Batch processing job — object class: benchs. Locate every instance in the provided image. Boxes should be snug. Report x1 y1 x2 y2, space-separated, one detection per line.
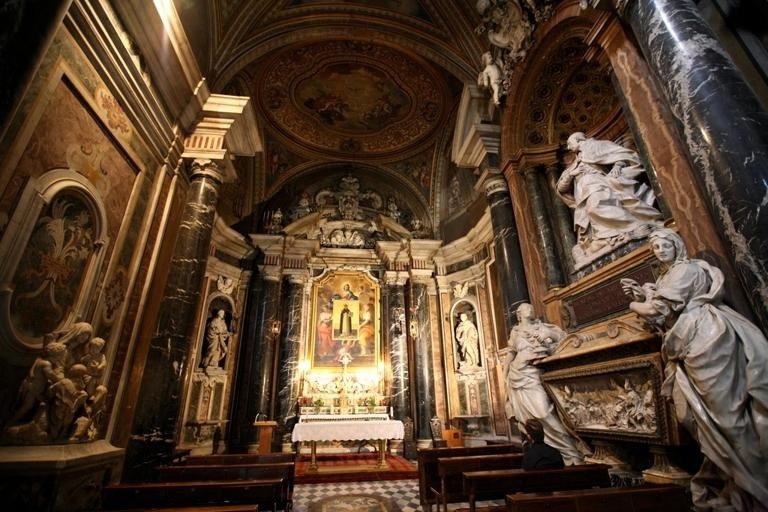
417 441 687 512
97 451 296 512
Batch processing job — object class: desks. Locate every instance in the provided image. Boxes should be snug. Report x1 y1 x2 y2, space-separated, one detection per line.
291 413 405 473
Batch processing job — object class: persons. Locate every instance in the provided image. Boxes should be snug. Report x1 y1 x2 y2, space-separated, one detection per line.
200 307 234 371
516 418 569 471
211 425 222 455
46 362 88 439
340 302 354 337
4 342 67 439
333 282 358 300
43 321 94 373
355 302 376 356
618 226 768 510
334 339 357 360
502 301 592 467
318 302 337 361
72 336 108 444
455 310 479 368
475 50 506 106
555 129 668 270
